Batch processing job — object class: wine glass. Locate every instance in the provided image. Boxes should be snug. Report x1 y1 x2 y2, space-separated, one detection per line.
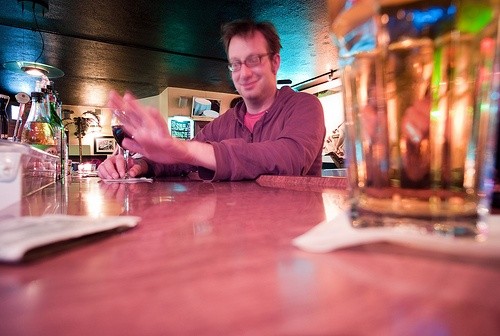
111 113 147 182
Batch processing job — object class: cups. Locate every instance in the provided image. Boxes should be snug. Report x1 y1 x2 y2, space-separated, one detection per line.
334 0 500 238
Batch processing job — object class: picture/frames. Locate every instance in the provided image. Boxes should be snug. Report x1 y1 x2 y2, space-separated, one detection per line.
94 136 117 154
191 96 222 118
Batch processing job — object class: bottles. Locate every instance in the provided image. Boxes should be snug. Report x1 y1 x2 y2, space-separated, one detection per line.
23 84 69 180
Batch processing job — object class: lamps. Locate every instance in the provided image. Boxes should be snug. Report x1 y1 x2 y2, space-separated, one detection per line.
327 69 334 81
2 0 65 79
72 116 94 164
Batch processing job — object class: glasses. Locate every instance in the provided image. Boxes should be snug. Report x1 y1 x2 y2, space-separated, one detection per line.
226 52 270 72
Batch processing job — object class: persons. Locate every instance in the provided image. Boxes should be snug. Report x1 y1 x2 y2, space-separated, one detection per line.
96 18 326 182
